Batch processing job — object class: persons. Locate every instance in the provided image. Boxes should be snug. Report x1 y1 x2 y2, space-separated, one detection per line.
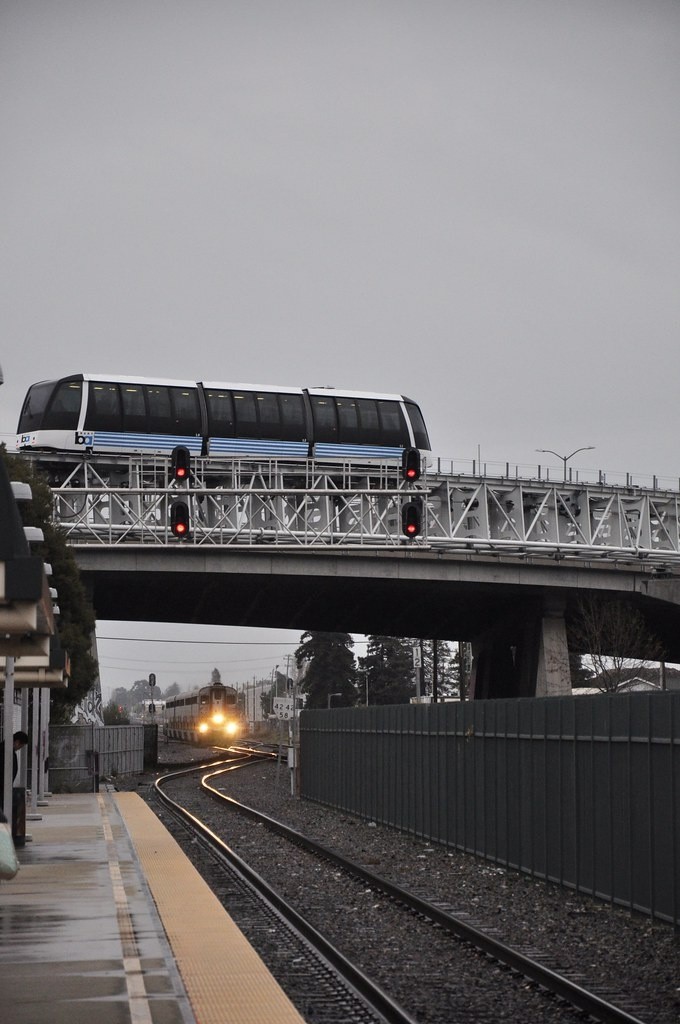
0 731 31 786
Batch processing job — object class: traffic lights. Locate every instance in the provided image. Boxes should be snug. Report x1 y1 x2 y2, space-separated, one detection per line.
401 446 420 483
403 503 422 537
170 445 192 482
119 706 123 714
171 500 189 536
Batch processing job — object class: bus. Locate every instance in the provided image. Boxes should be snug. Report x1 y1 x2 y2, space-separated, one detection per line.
15 372 435 471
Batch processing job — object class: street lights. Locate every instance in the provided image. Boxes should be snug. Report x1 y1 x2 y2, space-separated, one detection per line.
275 664 279 698
535 446 595 482
327 691 342 710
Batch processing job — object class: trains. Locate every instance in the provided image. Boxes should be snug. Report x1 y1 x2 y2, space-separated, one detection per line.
162 683 241 747
